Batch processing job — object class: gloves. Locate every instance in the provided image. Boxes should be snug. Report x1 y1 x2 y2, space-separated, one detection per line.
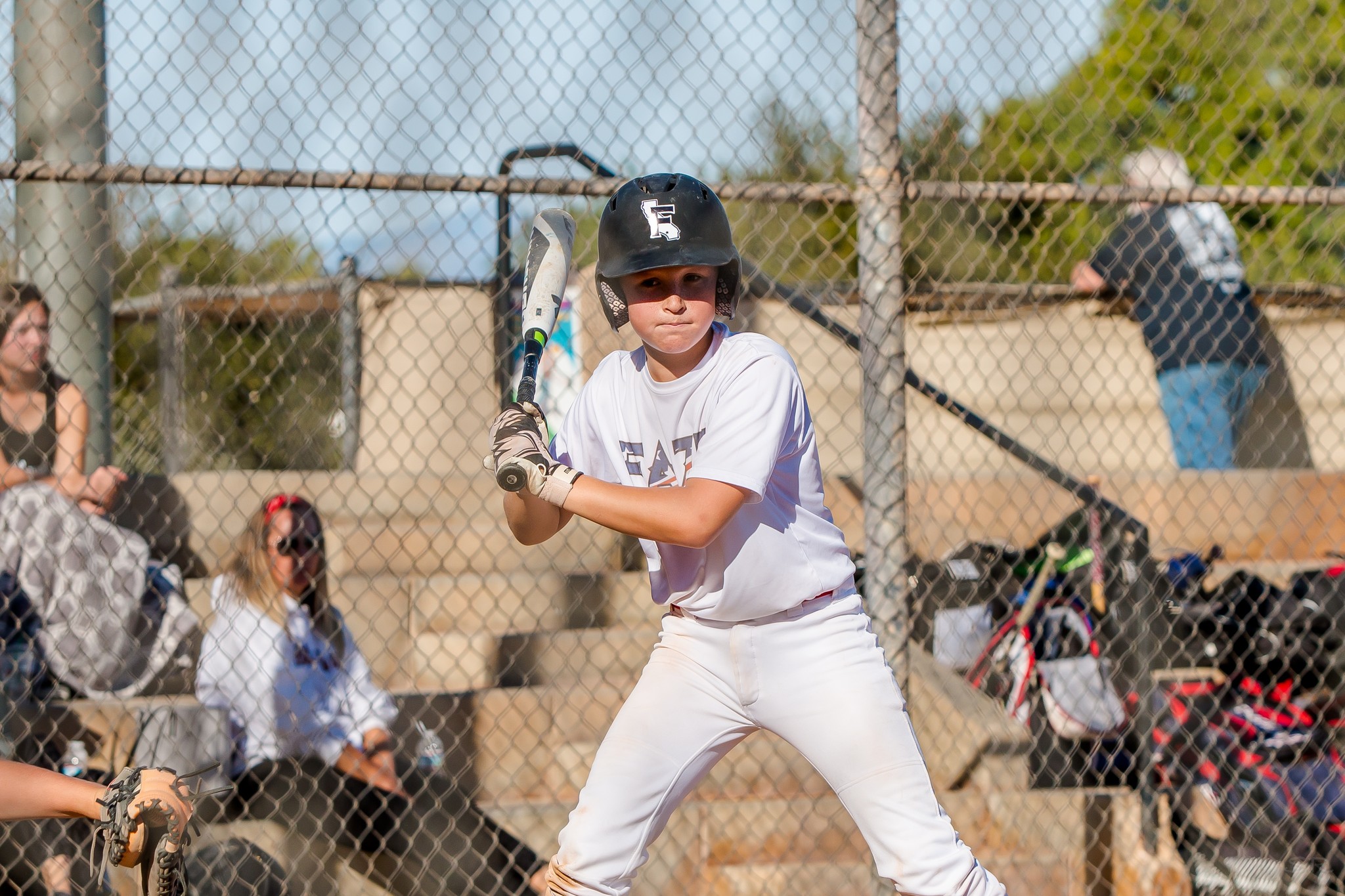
482 427 583 522
491 399 549 423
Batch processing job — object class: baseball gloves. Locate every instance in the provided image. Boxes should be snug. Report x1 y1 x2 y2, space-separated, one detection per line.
87 760 236 896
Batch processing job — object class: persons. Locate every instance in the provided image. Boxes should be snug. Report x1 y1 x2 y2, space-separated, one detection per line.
193 493 551 896
492 171 1005 896
0 280 196 896
1071 146 1280 467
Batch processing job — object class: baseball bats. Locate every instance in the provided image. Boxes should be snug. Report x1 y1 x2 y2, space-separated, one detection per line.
494 207 578 494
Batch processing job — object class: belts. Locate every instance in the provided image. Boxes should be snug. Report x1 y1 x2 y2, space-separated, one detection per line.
669 588 834 618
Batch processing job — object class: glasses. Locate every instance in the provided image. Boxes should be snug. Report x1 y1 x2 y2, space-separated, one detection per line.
270 534 325 557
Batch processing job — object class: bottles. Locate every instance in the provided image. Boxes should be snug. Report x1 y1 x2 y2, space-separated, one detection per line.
417 730 443 772
64 740 88 779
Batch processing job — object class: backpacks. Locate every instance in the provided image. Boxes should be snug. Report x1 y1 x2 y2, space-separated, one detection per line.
881 472 1336 876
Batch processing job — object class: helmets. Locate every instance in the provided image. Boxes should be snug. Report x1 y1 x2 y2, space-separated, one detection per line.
594 173 744 334
